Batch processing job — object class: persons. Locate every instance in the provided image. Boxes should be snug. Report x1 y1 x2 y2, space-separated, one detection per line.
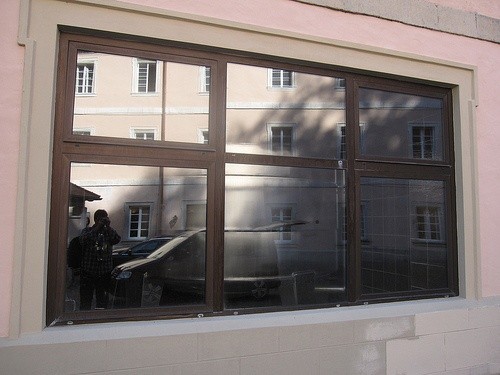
77 208 122 309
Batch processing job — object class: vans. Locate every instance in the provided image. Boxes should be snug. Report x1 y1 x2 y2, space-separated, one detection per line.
108 220 307 307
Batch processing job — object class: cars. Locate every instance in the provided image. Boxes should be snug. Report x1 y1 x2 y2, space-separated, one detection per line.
112 237 173 272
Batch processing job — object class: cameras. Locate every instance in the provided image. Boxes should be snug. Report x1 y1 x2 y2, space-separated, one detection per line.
100 217 109 224
